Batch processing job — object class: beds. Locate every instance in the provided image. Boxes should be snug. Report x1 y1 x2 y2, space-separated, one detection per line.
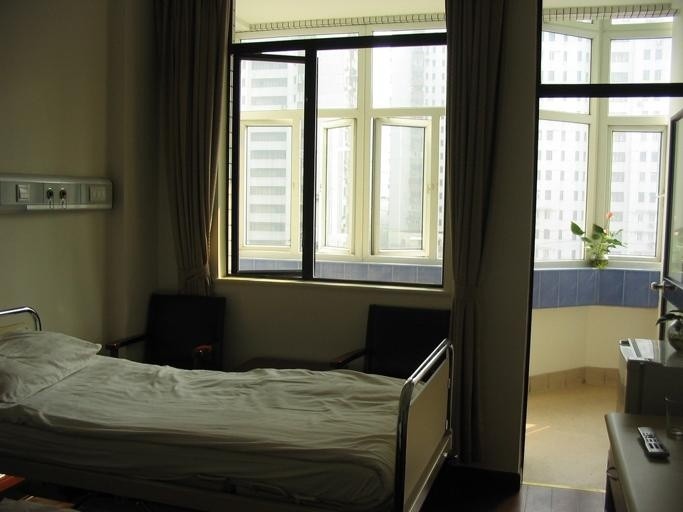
0 306 457 511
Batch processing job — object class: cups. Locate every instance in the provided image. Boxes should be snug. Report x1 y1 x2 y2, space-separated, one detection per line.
664 393 682 434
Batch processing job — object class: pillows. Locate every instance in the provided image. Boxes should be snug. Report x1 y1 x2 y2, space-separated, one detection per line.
0 329 103 409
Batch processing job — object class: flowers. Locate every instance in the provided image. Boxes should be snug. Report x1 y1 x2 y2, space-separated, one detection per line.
570 210 630 254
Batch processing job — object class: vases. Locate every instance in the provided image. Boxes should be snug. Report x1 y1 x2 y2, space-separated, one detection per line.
588 251 609 270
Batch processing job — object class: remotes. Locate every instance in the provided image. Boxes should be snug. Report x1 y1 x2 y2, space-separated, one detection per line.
637 426 669 458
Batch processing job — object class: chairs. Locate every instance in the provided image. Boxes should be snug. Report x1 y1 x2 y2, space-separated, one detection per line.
105 292 227 371
328 304 451 384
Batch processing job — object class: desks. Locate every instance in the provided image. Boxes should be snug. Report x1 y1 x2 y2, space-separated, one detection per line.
603 412 682 512
